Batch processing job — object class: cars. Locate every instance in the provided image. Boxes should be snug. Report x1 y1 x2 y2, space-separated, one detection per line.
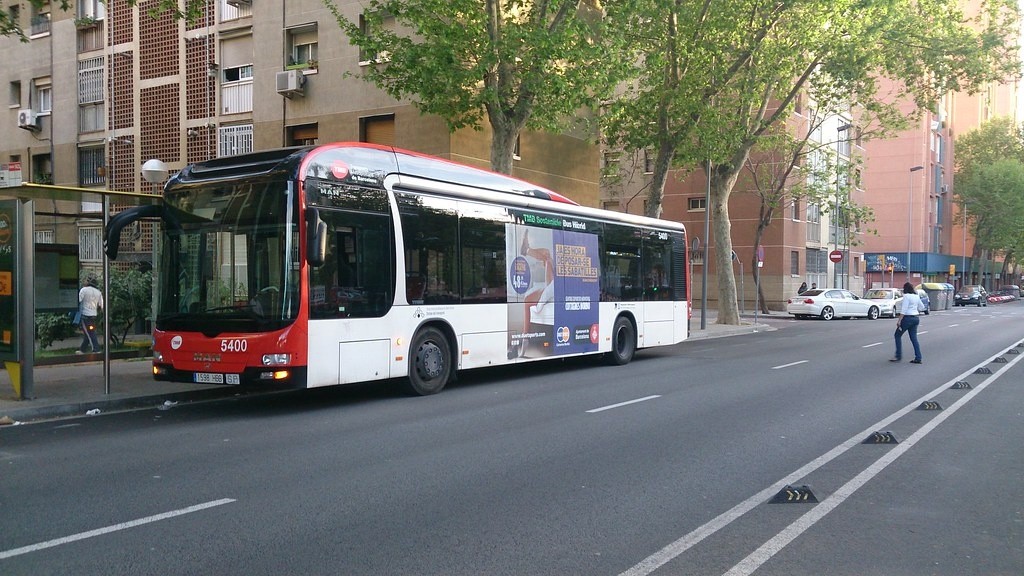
914 289 932 315
787 288 882 320
862 287 903 318
955 285 989 307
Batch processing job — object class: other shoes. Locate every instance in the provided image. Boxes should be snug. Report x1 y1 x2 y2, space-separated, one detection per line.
889 356 899 362
92 350 101 353
76 350 86 355
910 358 921 363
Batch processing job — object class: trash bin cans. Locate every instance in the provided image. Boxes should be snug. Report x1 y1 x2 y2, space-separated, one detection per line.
915 283 955 311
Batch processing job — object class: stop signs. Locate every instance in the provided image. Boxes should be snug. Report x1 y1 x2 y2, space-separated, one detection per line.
830 250 842 263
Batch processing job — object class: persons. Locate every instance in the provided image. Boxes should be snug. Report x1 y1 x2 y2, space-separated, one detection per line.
798 282 807 294
518 228 554 358
888 282 926 364
74 273 104 355
811 282 818 290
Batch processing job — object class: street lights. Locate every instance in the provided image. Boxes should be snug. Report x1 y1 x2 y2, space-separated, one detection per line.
907 165 925 283
833 123 853 287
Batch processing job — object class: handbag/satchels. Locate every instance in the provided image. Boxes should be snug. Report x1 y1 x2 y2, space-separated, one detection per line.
71 311 81 326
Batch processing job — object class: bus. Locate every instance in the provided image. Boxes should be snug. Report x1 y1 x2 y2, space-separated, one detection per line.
102 139 692 396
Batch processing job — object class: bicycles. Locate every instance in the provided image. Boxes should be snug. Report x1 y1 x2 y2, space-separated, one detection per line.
987 291 1015 304
